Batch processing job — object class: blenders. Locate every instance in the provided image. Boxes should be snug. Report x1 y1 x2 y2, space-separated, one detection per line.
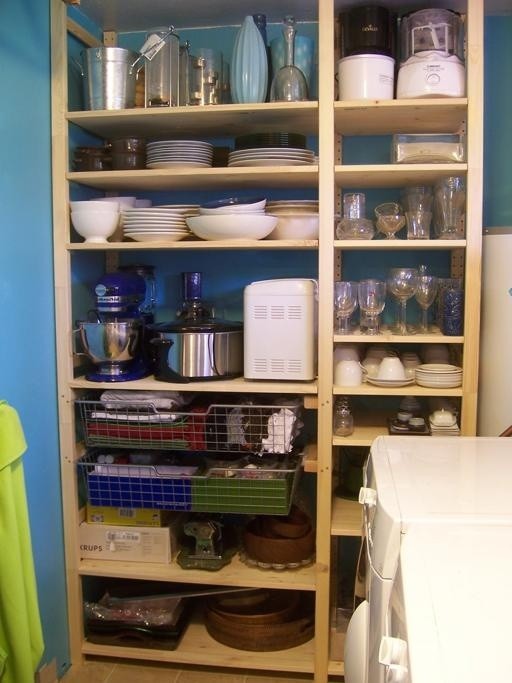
333 6 395 102
395 9 467 98
72 263 154 376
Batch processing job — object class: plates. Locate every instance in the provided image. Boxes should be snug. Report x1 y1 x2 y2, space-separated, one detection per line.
144 139 216 169
223 132 317 167
121 203 200 242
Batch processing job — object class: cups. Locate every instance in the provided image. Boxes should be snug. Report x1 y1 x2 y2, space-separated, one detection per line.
442 287 463 336
333 344 462 388
388 395 457 432
333 175 465 240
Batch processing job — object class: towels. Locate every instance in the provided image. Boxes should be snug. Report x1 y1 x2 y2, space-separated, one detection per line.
0 401 44 683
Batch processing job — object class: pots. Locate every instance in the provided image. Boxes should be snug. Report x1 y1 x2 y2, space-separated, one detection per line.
145 298 246 383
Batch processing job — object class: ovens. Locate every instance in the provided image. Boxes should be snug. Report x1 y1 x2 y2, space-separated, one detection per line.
344 434 512 683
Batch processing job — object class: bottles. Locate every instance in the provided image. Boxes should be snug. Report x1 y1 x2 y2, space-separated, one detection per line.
333 397 355 435
132 11 316 106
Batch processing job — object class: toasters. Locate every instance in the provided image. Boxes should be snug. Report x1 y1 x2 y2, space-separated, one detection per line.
240 277 317 383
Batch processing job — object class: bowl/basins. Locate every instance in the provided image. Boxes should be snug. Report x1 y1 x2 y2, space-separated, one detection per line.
242 503 314 564
70 196 154 242
186 195 323 242
73 136 146 170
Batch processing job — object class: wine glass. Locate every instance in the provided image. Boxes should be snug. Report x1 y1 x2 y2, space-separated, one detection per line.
330 265 440 334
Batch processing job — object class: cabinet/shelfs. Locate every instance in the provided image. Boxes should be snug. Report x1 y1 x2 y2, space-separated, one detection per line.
48 0 484 683
